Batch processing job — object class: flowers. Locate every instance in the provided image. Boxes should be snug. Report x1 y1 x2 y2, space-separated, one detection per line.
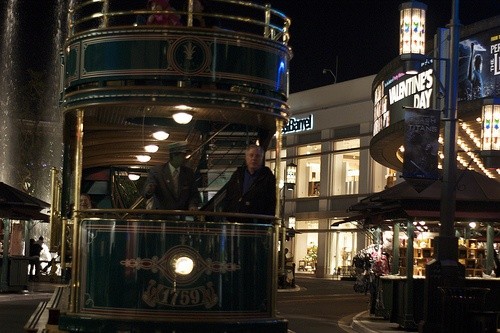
352 245 391 277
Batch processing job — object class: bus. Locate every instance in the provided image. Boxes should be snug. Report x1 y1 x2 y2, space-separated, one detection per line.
57 0 293 333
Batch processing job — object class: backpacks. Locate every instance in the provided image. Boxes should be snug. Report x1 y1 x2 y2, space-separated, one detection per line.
430 261 470 318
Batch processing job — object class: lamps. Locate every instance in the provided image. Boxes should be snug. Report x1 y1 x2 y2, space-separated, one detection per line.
171 111 193 125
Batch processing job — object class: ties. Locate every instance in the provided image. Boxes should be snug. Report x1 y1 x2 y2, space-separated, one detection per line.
173 168 179 197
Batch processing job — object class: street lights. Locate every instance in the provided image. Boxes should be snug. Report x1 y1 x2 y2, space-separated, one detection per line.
282 163 298 288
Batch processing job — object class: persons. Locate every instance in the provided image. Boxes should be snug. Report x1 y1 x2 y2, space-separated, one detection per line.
147 0 182 26
279 248 292 268
80 194 92 209
472 54 482 97
179 0 206 27
143 142 201 219
224 144 276 226
29 236 43 275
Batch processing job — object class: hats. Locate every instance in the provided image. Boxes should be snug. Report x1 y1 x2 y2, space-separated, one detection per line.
167 141 192 154
